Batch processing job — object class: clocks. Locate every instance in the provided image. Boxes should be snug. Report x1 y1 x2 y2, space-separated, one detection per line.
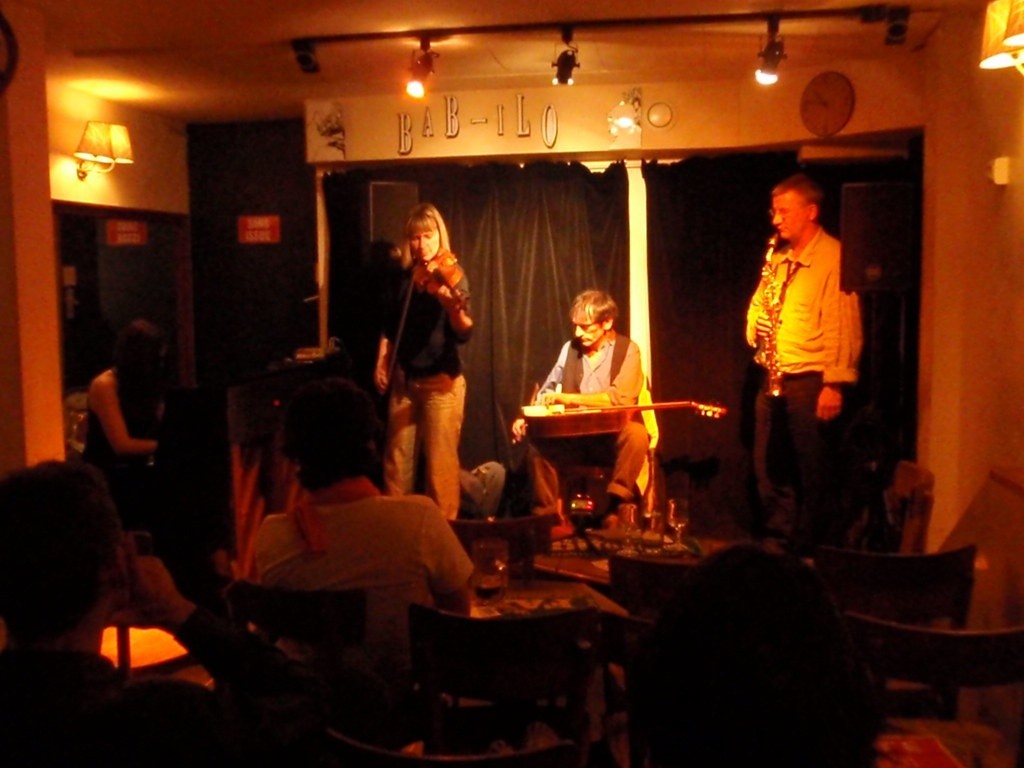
799 72 855 138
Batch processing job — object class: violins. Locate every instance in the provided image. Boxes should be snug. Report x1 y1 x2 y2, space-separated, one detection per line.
412 247 470 314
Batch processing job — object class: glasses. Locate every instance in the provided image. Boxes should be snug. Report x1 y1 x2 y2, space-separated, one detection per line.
768 204 809 216
569 320 597 330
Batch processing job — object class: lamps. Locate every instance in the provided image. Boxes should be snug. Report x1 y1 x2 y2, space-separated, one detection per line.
73 121 135 181
404 36 439 97
551 35 581 85
603 91 641 133
754 20 786 85
976 0 1024 75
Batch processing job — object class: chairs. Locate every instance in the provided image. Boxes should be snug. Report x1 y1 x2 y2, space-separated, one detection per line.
210 513 1024 768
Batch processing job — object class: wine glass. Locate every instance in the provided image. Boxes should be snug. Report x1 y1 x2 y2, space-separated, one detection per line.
668 499 689 550
618 503 639 556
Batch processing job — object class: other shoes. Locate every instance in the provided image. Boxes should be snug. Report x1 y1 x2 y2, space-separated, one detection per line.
548 520 576 541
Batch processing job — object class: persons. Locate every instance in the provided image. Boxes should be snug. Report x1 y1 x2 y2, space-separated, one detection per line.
512 290 659 528
247 378 482 687
0 461 321 768
83 321 167 474
375 205 472 520
628 544 889 768
745 174 862 542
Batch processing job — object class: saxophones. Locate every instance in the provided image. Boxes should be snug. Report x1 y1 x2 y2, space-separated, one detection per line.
753 232 785 402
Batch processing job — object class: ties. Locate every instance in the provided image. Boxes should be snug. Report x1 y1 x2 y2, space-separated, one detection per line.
776 260 801 326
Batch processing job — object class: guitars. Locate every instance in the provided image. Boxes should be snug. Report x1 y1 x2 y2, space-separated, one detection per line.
524 401 729 439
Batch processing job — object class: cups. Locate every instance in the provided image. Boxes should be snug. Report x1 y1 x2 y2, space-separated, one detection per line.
639 510 665 553
473 541 508 605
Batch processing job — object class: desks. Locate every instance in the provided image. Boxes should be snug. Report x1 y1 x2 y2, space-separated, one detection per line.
456 581 629 753
530 537 736 589
